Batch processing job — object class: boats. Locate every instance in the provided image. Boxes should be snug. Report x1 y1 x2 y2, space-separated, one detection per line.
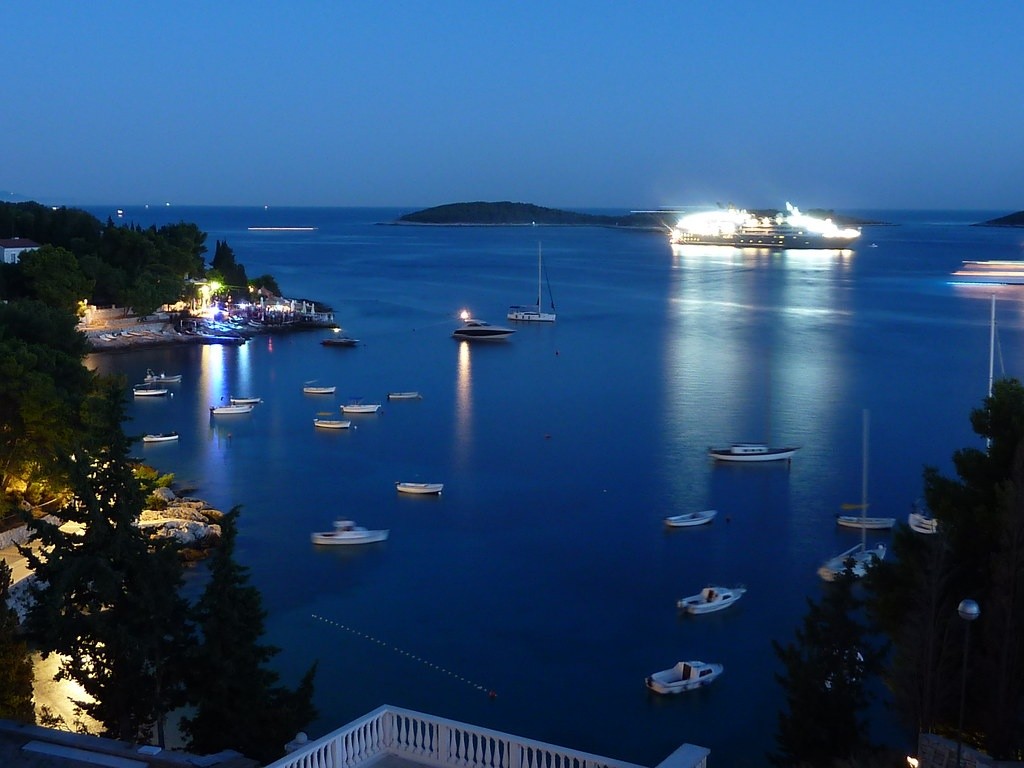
451 318 518 343
706 442 803 462
645 660 724 694
834 511 897 529
663 509 718 526
339 404 381 413
907 512 939 535
209 404 255 414
387 392 418 399
395 481 443 494
313 419 351 428
142 431 179 441
303 387 337 394
135 383 151 390
143 368 182 383
322 336 360 346
667 201 862 250
133 388 169 395
676 587 748 615
230 396 261 403
949 260 1024 284
310 520 391 545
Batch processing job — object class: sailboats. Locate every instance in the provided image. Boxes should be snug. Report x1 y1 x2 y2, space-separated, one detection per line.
817 410 887 582
507 241 557 322
